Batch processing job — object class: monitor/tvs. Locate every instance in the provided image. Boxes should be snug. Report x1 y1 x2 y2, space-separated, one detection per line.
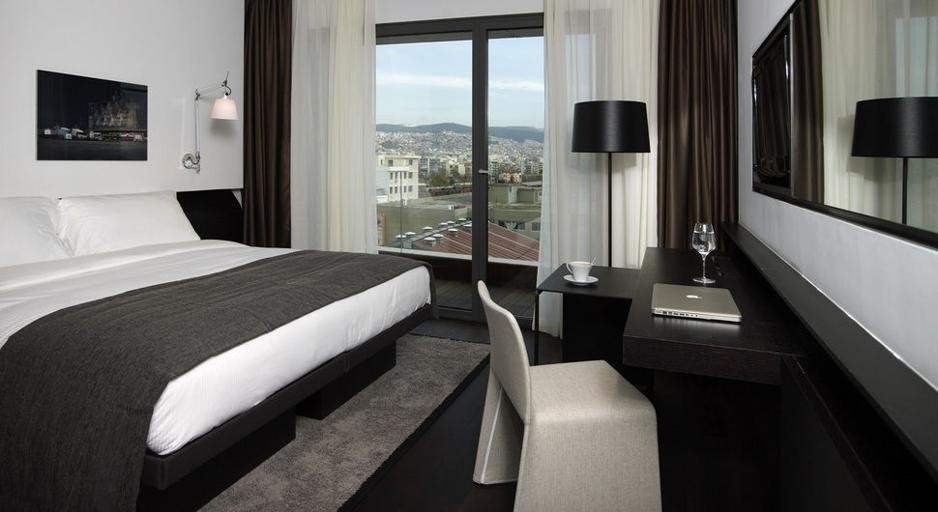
752 33 791 189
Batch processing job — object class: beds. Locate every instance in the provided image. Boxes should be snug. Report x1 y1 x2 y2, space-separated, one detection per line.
0 189 437 512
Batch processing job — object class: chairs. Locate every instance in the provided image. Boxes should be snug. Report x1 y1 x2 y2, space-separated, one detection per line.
472 280 662 512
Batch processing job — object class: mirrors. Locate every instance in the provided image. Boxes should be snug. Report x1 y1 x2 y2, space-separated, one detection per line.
752 0 938 249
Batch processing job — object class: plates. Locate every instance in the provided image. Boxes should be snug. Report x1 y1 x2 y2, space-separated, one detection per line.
563 274 600 287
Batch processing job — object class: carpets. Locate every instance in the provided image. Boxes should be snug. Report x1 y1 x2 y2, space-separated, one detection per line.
195 332 491 512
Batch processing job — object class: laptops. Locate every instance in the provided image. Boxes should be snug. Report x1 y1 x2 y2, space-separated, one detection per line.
651 283 742 324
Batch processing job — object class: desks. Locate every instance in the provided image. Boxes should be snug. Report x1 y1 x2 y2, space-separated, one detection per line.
533 246 805 386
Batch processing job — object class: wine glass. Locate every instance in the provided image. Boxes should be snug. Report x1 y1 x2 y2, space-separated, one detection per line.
692 222 717 284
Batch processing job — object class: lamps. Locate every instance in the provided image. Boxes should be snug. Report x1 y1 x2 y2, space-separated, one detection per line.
851 95 938 225
182 71 239 172
571 99 651 267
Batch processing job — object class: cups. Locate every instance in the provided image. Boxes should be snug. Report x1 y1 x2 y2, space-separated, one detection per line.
565 261 593 281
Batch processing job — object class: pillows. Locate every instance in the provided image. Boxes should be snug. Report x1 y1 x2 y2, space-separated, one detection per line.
0 196 70 267
55 191 201 258
